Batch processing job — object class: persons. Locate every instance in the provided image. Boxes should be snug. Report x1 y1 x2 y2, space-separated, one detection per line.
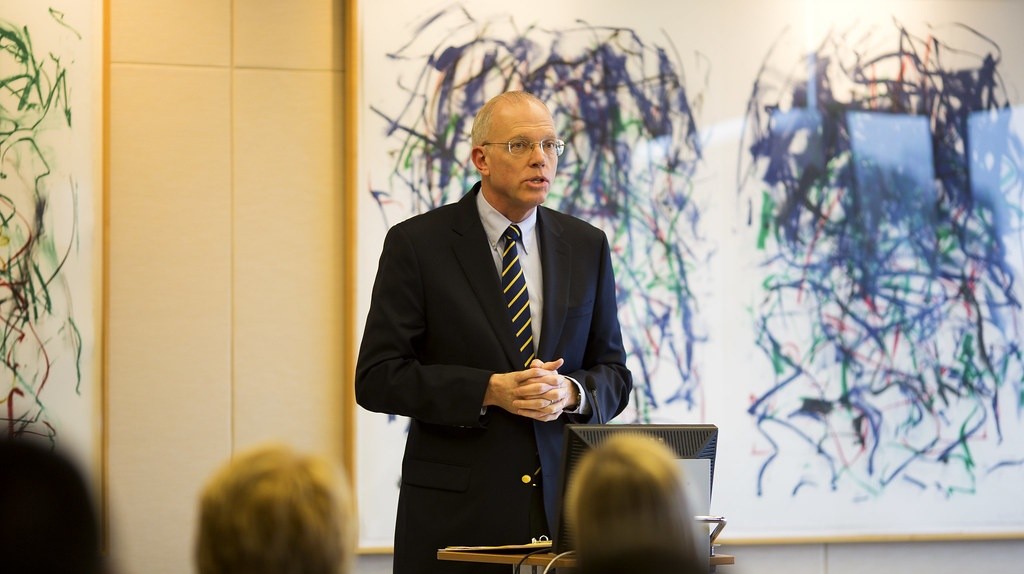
566 432 712 573
190 440 358 573
350 90 633 574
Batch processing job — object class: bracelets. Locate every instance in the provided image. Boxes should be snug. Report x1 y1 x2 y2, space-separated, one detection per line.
563 378 581 411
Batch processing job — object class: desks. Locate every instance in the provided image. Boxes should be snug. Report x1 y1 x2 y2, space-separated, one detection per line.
437 546 740 571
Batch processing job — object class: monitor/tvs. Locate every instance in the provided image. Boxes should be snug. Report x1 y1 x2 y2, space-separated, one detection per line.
551 423 719 555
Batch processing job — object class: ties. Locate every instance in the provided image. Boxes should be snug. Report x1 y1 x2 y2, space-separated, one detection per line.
499 224 537 370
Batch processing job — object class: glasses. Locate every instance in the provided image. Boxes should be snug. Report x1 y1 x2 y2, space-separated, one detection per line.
481 137 567 158
